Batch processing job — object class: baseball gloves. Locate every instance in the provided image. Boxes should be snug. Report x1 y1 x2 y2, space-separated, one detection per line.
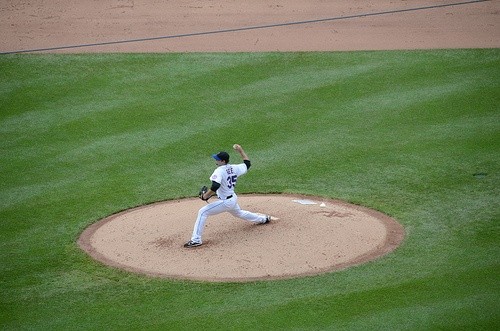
198 185 212 201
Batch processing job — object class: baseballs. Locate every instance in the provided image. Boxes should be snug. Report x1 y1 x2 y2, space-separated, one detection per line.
232 143 239 150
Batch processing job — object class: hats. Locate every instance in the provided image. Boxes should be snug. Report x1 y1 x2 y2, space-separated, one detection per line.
212 151 229 161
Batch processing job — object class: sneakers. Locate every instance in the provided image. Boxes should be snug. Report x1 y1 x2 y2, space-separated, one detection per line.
266 217 272 223
183 241 202 247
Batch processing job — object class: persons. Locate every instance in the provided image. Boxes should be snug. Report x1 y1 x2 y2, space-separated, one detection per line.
183 143 274 249
